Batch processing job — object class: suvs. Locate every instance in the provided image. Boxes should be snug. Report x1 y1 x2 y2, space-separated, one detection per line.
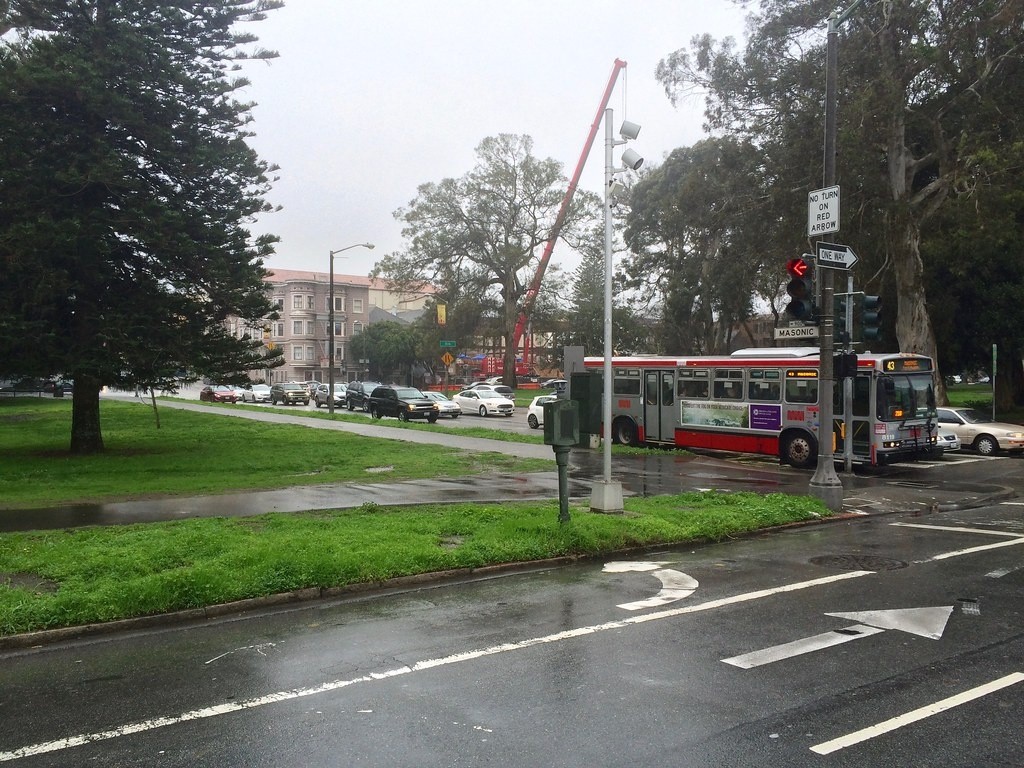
314 384 348 408
368 385 439 423
345 380 382 412
270 382 310 405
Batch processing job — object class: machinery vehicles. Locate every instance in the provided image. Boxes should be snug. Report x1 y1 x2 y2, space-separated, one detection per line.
480 58 626 380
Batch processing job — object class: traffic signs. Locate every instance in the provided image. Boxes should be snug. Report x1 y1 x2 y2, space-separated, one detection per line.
816 241 859 271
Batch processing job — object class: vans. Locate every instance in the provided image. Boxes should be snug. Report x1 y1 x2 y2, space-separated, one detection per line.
470 385 515 403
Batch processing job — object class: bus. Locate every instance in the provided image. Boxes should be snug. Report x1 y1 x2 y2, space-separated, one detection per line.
584 347 938 469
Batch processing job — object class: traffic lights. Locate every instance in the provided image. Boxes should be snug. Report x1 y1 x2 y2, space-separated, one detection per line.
785 259 812 318
863 294 884 343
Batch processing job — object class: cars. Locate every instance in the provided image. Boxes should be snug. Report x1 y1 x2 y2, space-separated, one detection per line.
460 382 490 390
14 378 73 393
451 390 515 417
200 380 321 404
540 379 558 388
920 429 961 459
527 396 559 429
419 392 461 419
916 408 1024 456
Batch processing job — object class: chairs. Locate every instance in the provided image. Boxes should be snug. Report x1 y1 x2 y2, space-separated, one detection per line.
612 378 819 406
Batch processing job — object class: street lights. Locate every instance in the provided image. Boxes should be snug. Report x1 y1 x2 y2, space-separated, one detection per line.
329 243 375 413
